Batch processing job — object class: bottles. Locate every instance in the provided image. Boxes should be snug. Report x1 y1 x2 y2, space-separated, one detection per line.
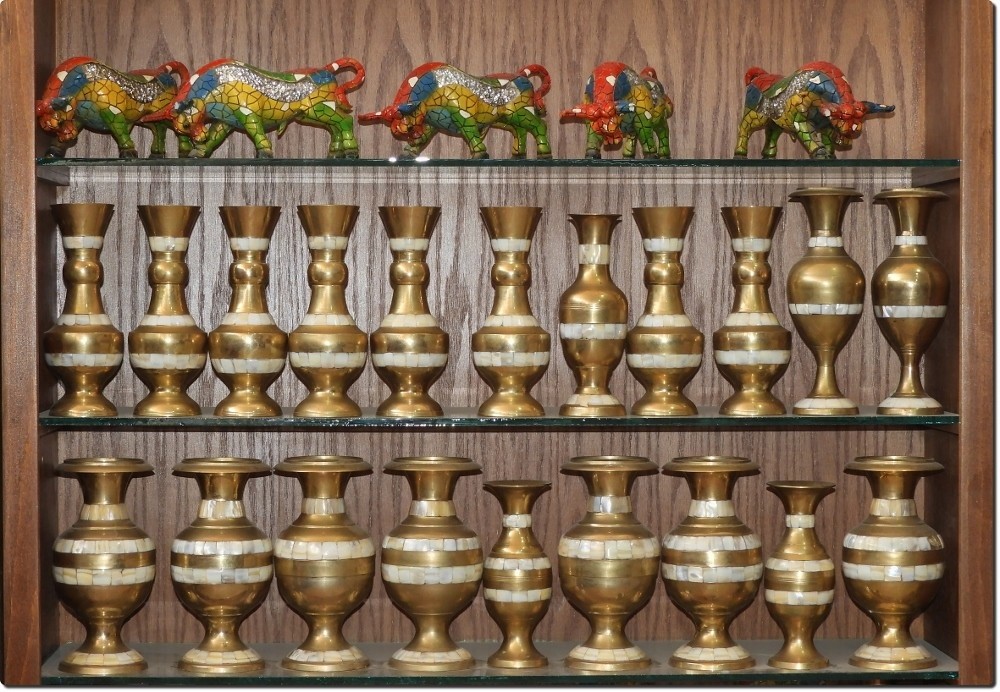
787 188 950 415
840 456 947 670
557 456 764 671
209 206 288 417
560 214 628 417
53 459 157 674
764 481 835 670
625 207 792 415
288 205 443 417
472 207 551 419
128 206 208 417
483 480 553 668
170 455 376 672
380 456 483 672
43 203 124 416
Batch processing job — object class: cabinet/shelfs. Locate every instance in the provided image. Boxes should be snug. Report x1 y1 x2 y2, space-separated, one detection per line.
39 158 963 687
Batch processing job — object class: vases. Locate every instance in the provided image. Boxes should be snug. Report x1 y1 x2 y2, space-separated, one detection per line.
53 455 947 674
49 186 949 421
